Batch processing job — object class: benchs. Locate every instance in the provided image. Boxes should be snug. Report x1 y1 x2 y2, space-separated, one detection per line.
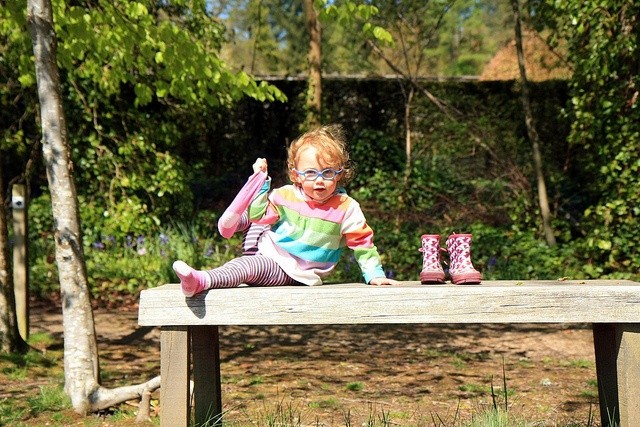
136 278 640 427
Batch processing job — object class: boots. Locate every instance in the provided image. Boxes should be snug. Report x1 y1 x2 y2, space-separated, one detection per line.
420 235 445 283
446 234 480 284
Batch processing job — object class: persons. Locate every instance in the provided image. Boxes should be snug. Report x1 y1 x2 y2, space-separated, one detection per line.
172 127 401 299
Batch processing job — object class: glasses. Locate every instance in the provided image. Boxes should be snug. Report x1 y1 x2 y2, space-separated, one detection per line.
293 169 345 181
